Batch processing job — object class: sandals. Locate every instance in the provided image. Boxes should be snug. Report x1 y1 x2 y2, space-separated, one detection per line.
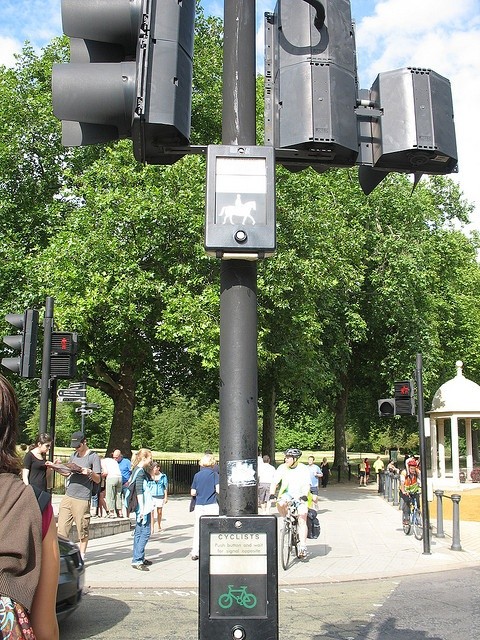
119 514 123 518
107 514 115 519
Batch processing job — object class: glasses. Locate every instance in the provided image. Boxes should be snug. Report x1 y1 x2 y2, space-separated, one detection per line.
74 444 84 448
38 442 52 448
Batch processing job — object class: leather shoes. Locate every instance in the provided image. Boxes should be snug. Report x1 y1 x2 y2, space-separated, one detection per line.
158 528 161 532
191 555 199 560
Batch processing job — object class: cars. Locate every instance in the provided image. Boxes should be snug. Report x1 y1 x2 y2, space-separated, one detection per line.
56 533 84 622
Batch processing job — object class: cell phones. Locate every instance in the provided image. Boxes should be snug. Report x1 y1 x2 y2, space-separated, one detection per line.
148 463 155 469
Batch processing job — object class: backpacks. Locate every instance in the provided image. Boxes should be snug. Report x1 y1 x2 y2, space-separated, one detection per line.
307 507 320 539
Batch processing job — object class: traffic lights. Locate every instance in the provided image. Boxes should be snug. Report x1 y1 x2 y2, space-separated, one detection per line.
379 380 415 416
264 0 457 195
49 333 77 378
52 1 195 165
2 310 37 378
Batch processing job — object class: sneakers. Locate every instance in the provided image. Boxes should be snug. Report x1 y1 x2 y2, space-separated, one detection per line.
143 559 153 565
132 563 149 571
403 518 409 525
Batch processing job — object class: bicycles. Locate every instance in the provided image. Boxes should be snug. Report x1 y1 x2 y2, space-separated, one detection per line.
269 496 306 569
402 492 425 540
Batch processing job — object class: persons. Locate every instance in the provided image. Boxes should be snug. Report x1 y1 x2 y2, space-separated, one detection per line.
386 457 397 474
1 374 60 640
306 455 324 504
125 447 154 571
364 455 371 485
268 448 309 571
257 454 276 509
189 454 219 561
399 458 421 524
149 460 169 535
319 457 333 489
45 429 101 562
111 448 131 520
21 433 61 493
357 458 368 488
97 452 124 520
373 455 385 489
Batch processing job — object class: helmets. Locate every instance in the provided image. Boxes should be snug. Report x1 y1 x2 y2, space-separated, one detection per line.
407 460 417 475
284 447 302 467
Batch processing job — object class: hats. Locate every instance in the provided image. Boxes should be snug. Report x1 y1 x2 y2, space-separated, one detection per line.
70 431 85 448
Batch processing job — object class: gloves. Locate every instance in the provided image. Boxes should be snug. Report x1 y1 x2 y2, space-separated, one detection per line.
269 494 277 500
299 495 309 502
